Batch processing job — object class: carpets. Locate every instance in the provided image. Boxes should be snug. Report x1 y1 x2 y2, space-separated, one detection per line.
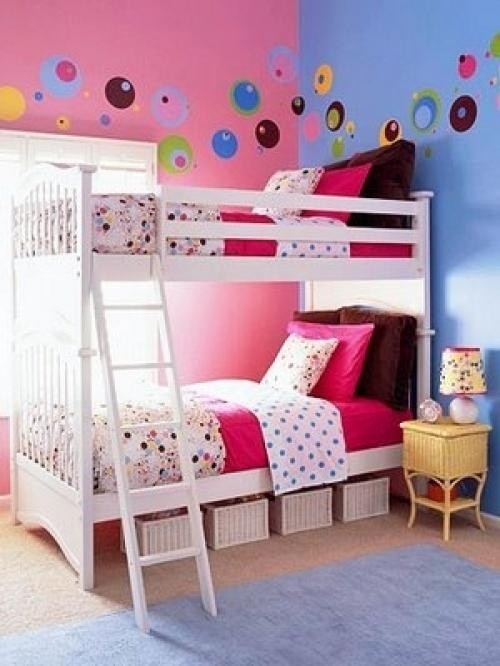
0 539 500 666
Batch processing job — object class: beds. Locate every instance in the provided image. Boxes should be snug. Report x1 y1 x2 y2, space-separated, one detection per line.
8 159 433 590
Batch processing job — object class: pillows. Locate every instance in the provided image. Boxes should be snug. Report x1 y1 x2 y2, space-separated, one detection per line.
285 318 377 400
322 159 349 172
259 332 339 395
292 307 340 325
339 306 416 411
300 162 372 225
346 139 415 228
251 167 324 217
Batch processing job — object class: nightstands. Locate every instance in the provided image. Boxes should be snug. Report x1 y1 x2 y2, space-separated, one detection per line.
399 416 493 540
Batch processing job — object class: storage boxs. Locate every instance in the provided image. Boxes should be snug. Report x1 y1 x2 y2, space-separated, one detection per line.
332 469 391 524
119 507 205 557
200 493 269 551
266 485 333 536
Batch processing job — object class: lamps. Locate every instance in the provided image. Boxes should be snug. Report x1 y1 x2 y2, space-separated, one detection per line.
437 348 487 425
